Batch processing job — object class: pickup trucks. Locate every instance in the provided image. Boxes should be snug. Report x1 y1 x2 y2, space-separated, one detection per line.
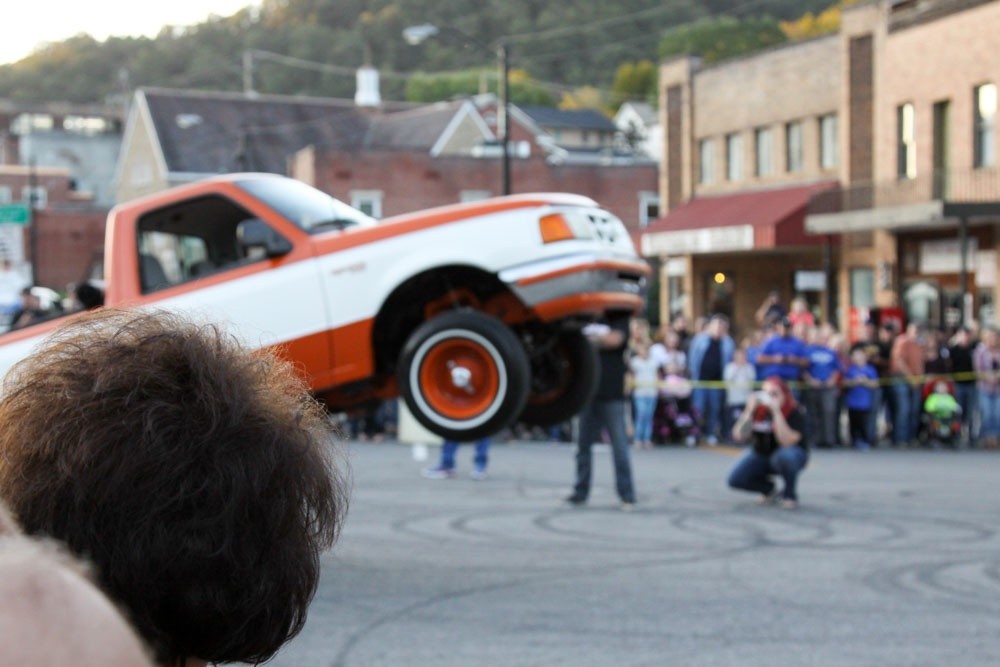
1 171 653 443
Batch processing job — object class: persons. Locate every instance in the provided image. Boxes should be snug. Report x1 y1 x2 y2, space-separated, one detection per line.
0 279 1000 667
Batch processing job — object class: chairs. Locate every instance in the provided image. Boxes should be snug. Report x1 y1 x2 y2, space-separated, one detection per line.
139 215 293 298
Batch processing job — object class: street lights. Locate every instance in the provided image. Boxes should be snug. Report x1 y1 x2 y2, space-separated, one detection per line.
402 22 513 194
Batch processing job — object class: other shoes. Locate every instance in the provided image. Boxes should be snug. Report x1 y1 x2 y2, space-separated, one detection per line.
781 499 794 509
760 486 778 504
